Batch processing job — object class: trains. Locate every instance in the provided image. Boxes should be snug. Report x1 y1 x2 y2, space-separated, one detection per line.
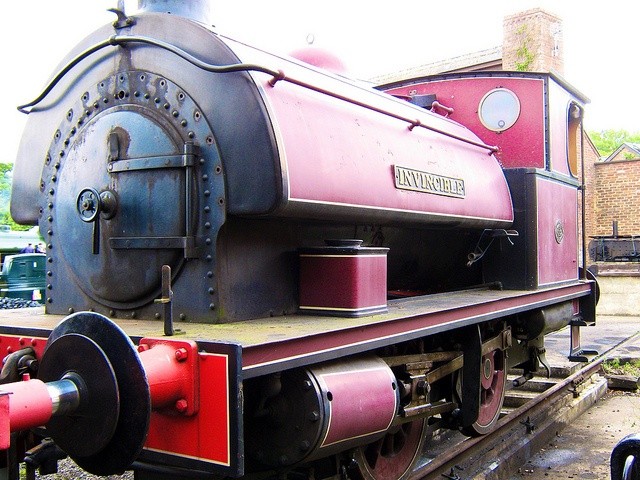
1 0 601 480
